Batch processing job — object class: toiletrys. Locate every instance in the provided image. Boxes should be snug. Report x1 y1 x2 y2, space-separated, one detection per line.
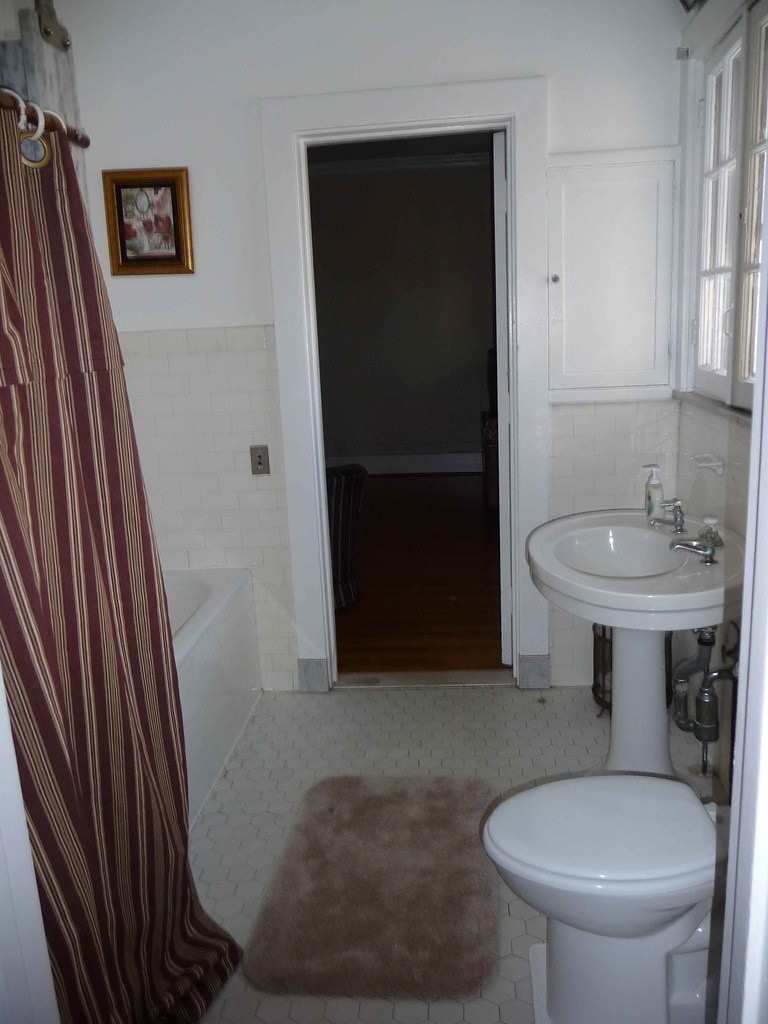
642 464 666 524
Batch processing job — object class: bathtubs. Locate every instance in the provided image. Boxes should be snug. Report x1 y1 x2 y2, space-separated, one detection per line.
161 560 266 838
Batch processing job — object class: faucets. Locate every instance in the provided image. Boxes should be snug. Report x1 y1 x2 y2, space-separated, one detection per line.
669 528 720 567
647 497 690 535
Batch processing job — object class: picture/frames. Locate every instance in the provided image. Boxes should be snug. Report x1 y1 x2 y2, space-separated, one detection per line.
100 163 195 279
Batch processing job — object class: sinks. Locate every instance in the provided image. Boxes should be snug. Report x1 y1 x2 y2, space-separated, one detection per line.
525 502 750 635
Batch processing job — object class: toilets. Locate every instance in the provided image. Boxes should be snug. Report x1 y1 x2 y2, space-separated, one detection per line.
479 770 736 1024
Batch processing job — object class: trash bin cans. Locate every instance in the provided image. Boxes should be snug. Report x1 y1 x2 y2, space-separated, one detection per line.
592 622 675 719
